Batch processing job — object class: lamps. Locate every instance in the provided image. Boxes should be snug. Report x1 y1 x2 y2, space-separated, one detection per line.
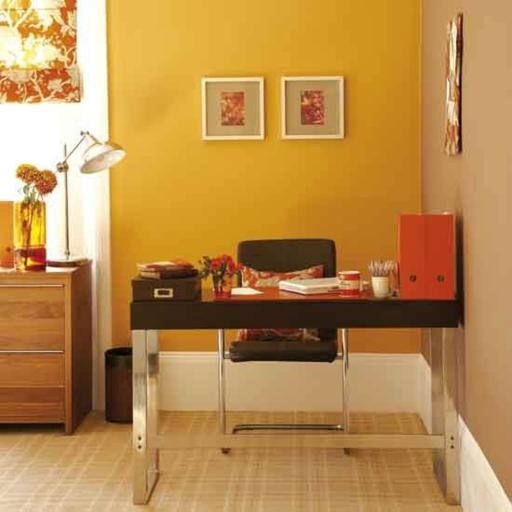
46 130 126 268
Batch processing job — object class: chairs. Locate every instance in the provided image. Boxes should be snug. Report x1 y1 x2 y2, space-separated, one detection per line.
218 238 350 455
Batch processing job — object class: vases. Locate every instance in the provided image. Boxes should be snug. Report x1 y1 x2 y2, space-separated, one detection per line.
213 276 232 298
13 202 47 271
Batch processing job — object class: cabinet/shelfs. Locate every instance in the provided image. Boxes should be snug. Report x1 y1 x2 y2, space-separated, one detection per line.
0 257 92 435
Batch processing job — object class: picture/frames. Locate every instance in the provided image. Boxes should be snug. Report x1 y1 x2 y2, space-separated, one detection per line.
280 76 345 139
201 77 264 140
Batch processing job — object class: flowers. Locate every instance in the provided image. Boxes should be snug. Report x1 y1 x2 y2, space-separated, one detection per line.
198 255 244 288
15 164 58 266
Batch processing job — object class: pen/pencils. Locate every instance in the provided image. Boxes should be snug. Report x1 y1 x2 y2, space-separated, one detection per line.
369 260 393 276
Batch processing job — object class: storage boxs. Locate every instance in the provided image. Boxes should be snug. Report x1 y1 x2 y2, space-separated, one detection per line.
397 212 456 300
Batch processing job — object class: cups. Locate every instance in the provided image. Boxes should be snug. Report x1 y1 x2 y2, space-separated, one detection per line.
370 276 388 297
338 270 361 298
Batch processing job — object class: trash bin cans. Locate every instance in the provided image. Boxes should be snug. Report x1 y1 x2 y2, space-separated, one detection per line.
104 347 133 423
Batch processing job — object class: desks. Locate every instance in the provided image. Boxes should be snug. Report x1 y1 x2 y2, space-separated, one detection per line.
130 286 460 505
